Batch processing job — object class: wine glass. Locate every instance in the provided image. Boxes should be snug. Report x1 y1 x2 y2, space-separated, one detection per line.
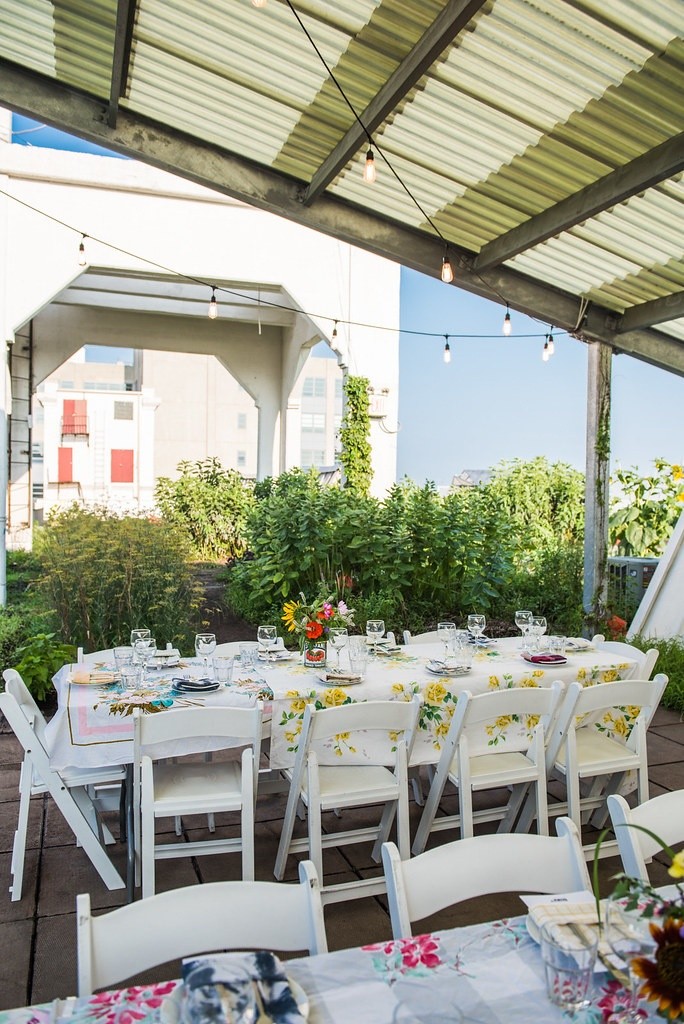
194 633 216 680
528 616 548 655
257 625 278 671
515 611 534 651
366 620 385 662
606 891 664 1024
468 614 486 655
131 629 152 675
437 622 456 664
328 628 348 672
133 637 157 688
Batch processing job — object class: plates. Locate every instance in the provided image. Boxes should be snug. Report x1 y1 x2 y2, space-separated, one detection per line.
524 657 568 666
159 975 310 1024
556 646 595 652
67 671 121 686
170 685 222 694
525 914 605 954
314 675 364 686
423 665 472 676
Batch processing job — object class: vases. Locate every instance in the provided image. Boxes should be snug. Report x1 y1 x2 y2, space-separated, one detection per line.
304 641 327 668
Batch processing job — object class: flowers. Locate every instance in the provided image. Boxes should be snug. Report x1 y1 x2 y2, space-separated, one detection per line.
594 822 684 1024
281 571 355 656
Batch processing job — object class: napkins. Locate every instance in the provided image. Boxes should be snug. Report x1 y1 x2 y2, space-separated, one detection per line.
182 950 306 1024
315 666 362 684
71 670 116 684
172 676 219 691
565 637 590 650
521 650 567 664
426 660 470 675
527 896 639 975
365 639 392 654
259 643 287 656
152 647 180 663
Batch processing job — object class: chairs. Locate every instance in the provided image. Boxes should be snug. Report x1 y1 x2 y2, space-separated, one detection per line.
0 629 684 1001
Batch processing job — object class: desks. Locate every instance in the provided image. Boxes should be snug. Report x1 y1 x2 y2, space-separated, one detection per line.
0 881 684 1024
42 636 640 904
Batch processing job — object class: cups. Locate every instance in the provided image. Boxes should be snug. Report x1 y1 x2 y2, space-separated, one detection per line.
548 635 567 657
212 656 235 686
239 642 260 674
540 919 599 1011
113 646 134 675
349 636 368 677
120 659 142 695
